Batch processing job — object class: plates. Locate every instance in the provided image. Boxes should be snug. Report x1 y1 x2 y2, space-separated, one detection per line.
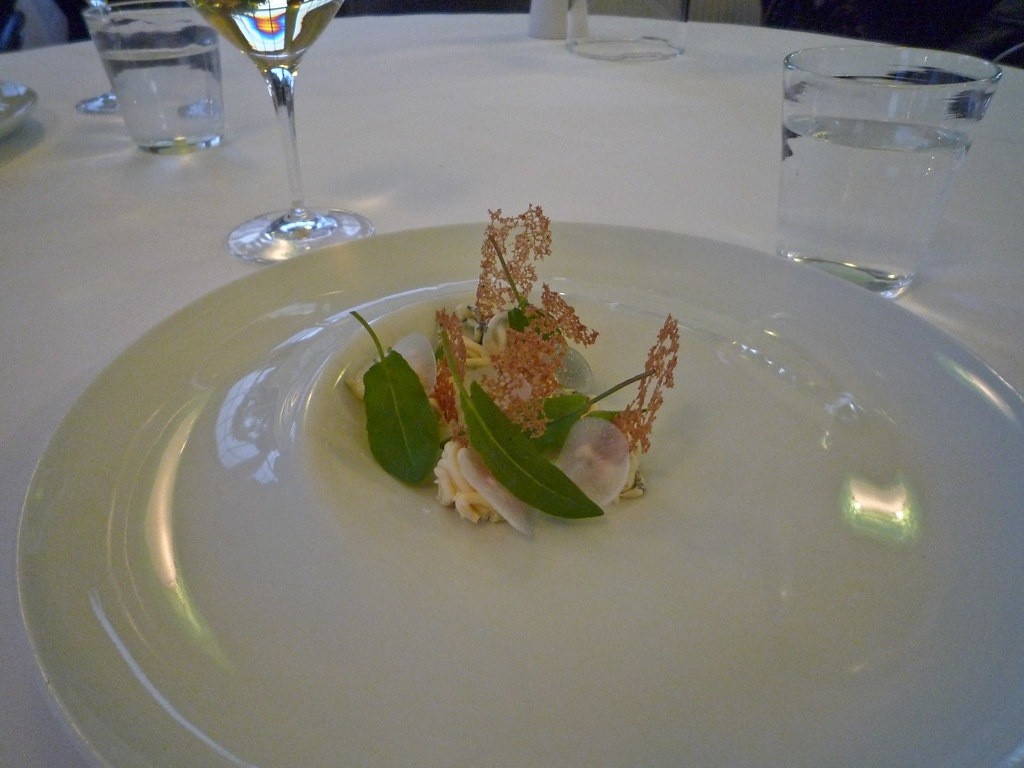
0 79 40 140
14 225 1024 768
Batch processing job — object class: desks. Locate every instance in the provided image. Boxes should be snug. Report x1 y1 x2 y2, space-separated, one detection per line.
0 13 1024 768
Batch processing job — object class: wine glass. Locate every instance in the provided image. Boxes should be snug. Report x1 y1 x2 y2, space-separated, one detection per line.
185 0 375 266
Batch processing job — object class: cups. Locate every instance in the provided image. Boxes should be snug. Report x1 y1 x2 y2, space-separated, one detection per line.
776 46 1003 297
81 0 225 155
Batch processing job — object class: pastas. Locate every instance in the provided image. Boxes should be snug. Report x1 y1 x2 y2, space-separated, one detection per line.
425 333 646 517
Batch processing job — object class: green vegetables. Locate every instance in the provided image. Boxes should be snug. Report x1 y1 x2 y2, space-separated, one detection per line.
347 232 656 519
